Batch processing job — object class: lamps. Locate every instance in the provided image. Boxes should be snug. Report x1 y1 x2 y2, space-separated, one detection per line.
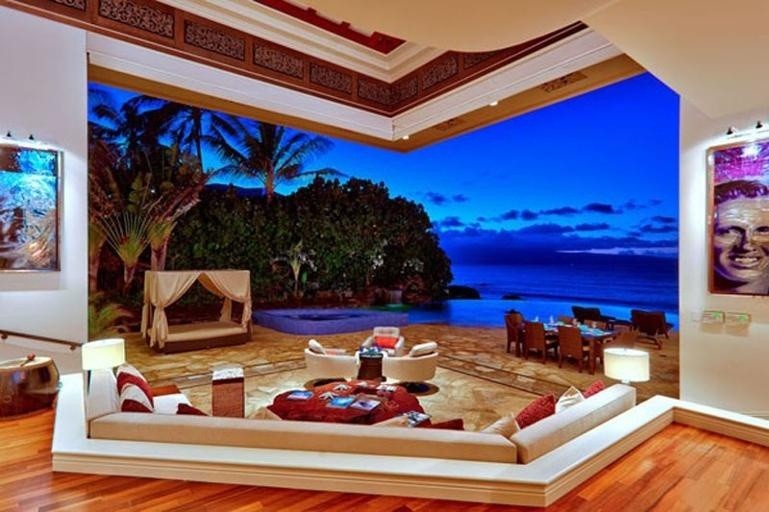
604 348 650 384
82 338 125 370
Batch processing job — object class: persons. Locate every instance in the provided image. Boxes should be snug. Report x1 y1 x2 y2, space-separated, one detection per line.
0 177 58 271
711 181 769 296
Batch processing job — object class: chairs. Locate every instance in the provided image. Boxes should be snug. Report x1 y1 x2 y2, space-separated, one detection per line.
304 338 357 379
381 341 439 388
504 306 674 375
360 326 405 357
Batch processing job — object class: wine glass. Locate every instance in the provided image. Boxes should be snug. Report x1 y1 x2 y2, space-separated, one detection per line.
591 321 597 333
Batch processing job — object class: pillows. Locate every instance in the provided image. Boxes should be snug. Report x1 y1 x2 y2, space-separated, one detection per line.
409 342 438 357
373 326 400 339
375 336 399 348
308 339 326 354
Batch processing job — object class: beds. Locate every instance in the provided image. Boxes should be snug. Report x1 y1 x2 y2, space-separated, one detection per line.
141 268 253 354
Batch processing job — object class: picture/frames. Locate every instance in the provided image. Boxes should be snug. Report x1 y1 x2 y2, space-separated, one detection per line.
703 138 769 299
0 143 65 273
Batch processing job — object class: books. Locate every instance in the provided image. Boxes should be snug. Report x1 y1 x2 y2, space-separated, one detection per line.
285 385 430 425
